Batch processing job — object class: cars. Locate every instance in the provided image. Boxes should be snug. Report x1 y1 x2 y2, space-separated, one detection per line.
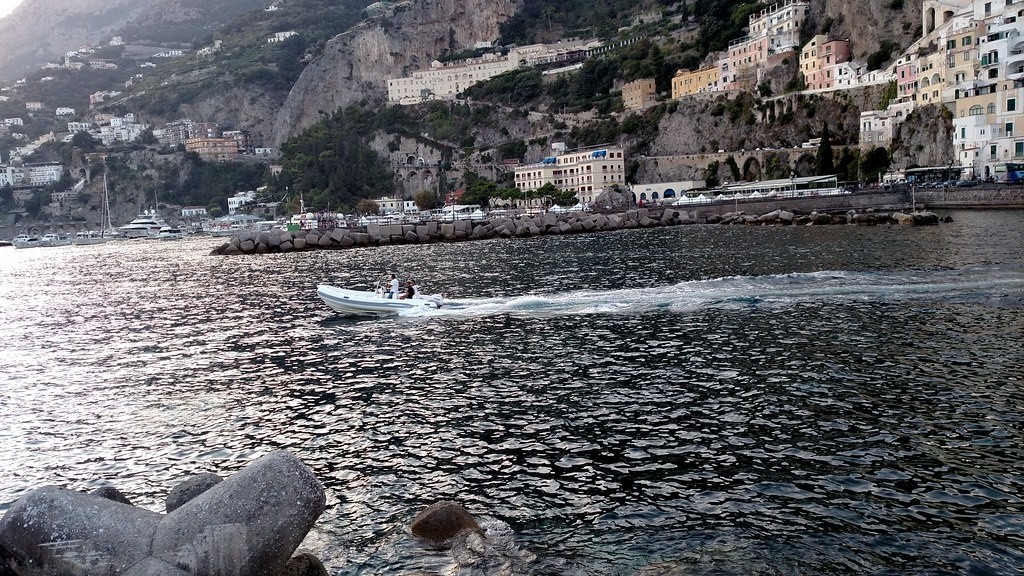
883 174 978 190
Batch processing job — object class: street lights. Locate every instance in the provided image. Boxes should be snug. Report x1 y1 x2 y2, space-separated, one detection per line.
946 159 954 188
887 163 897 190
450 190 456 220
788 171 798 197
625 183 636 210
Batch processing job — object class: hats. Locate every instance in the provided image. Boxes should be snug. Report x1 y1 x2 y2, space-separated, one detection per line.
406 280 411 282
390 274 397 278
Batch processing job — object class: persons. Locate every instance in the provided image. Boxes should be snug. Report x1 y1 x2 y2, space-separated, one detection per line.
400 280 418 299
384 274 399 299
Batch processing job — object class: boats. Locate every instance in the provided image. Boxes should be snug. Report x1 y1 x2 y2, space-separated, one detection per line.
0 171 268 250
317 284 439 313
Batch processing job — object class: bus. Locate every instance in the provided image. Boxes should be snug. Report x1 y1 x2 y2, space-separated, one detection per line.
991 160 1024 185
